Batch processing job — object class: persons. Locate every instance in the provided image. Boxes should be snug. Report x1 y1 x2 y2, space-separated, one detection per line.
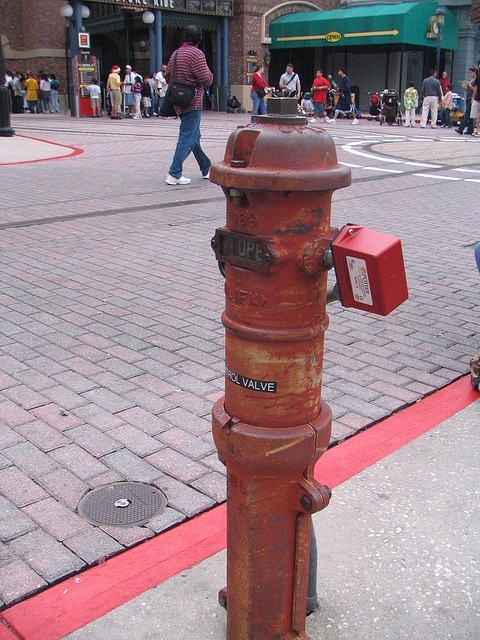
279 63 301 99
455 67 477 135
123 63 136 118
309 69 331 122
154 64 167 118
418 68 442 128
84 79 101 117
106 78 112 116
439 71 451 96
132 69 159 119
404 81 419 126
165 23 213 185
327 68 359 125
469 60 480 137
250 63 275 122
6 69 60 114
441 84 453 127
108 66 124 119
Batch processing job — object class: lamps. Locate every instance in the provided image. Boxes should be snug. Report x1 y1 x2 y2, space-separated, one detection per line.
60 4 74 24
81 5 90 19
142 11 155 34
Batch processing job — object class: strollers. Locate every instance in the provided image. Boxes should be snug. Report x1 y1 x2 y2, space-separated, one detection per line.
367 92 381 121
378 88 404 127
226 92 246 113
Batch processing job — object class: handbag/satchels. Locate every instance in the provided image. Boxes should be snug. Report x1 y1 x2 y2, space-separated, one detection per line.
257 88 265 99
164 81 195 109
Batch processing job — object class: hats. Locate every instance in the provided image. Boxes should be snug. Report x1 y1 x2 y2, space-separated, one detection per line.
111 65 120 72
126 65 132 70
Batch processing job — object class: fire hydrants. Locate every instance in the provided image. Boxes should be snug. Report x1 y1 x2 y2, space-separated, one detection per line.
204 89 352 639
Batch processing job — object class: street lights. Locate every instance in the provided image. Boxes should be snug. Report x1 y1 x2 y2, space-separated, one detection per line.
433 3 447 79
141 11 157 116
60 4 91 117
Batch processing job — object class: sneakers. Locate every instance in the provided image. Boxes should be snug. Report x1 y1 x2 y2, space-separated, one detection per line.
165 173 191 185
326 118 336 124
455 129 463 135
351 118 359 125
320 116 329 123
464 132 471 134
309 117 316 123
203 166 210 179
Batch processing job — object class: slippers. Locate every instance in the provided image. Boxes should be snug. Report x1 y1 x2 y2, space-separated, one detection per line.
471 132 477 136
474 134 480 138
